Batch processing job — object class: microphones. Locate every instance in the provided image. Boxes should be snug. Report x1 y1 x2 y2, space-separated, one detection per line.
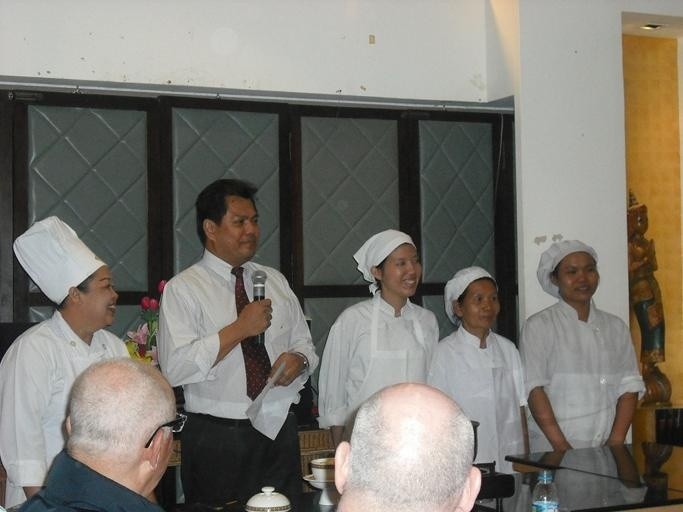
252 270 267 345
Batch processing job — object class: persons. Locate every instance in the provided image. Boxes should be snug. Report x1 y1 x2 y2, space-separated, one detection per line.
5 356 187 510
429 266 527 511
317 226 439 450
0 264 130 510
517 445 648 512
519 240 646 452
155 179 318 502
333 383 481 511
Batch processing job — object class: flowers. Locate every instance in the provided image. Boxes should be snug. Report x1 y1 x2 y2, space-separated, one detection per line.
127 279 167 349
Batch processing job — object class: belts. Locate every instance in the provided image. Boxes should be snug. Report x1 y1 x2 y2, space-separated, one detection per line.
208 415 251 427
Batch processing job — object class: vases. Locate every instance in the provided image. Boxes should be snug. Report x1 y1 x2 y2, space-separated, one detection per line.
146 346 158 364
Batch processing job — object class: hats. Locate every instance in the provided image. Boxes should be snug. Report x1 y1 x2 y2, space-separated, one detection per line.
13 215 108 306
443 265 498 327
537 240 598 298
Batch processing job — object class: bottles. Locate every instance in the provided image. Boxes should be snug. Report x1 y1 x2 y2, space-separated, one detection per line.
531 470 560 512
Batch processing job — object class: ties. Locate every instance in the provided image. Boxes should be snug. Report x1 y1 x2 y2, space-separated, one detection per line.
231 266 271 401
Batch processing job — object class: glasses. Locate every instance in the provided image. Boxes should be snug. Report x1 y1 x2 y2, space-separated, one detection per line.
145 412 188 449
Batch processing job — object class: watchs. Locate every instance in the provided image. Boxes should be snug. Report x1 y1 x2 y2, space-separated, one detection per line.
291 351 309 368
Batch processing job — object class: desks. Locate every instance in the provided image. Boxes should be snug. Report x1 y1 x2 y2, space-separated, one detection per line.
154 469 683 512
505 443 683 492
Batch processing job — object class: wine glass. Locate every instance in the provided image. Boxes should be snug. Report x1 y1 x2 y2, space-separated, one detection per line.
303 474 339 505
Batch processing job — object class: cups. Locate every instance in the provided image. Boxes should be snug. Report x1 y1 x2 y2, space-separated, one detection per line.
312 458 335 480
